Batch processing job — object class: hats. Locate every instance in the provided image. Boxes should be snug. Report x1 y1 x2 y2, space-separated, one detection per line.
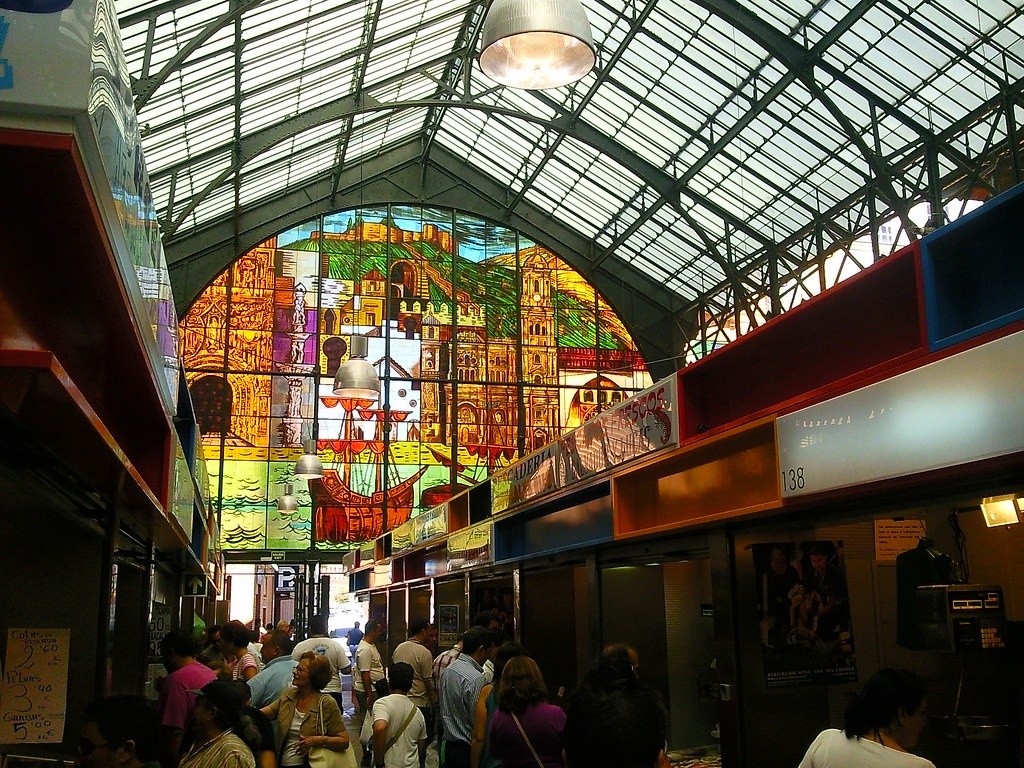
190 679 243 715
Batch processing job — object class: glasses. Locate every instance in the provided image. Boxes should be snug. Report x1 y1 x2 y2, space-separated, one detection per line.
77 737 109 757
293 664 310 673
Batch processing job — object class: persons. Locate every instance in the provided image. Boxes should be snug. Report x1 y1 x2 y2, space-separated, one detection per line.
759 543 850 641
797 668 937 768
77 585 673 768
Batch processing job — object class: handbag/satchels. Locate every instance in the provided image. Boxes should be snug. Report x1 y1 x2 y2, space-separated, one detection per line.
309 694 358 768
